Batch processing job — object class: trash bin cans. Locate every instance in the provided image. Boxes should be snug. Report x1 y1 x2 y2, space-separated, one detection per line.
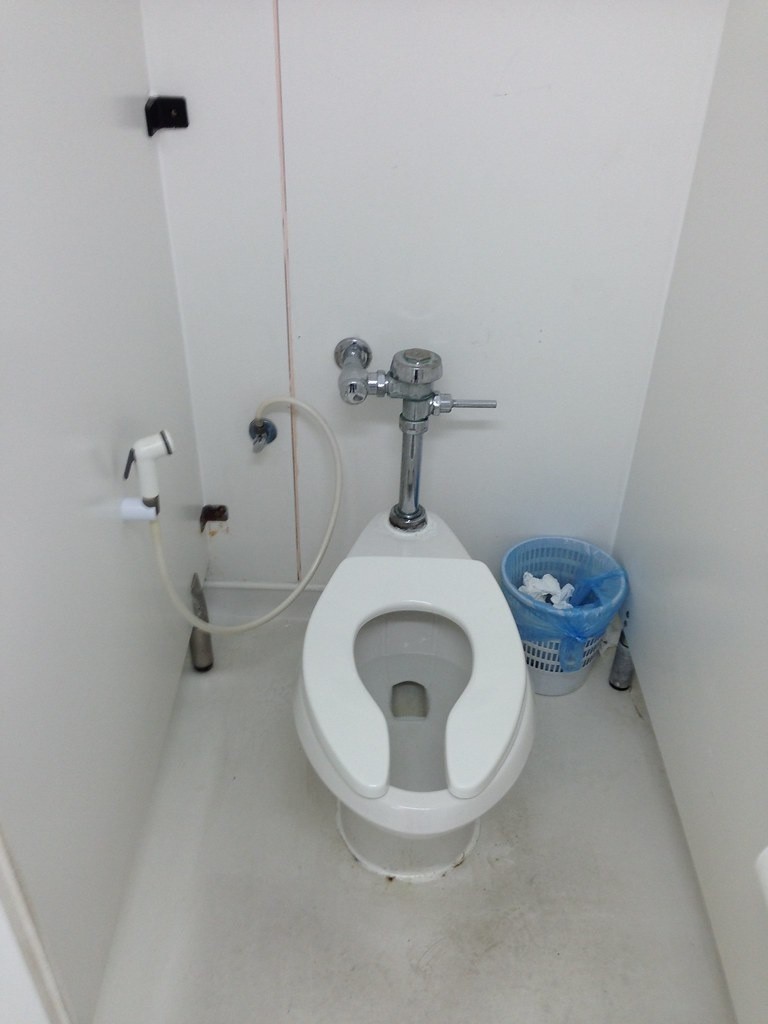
502 536 628 697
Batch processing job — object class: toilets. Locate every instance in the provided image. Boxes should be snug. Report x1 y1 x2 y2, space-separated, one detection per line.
300 507 532 878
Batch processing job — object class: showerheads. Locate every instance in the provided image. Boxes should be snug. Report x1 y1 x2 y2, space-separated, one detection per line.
124 431 174 514
